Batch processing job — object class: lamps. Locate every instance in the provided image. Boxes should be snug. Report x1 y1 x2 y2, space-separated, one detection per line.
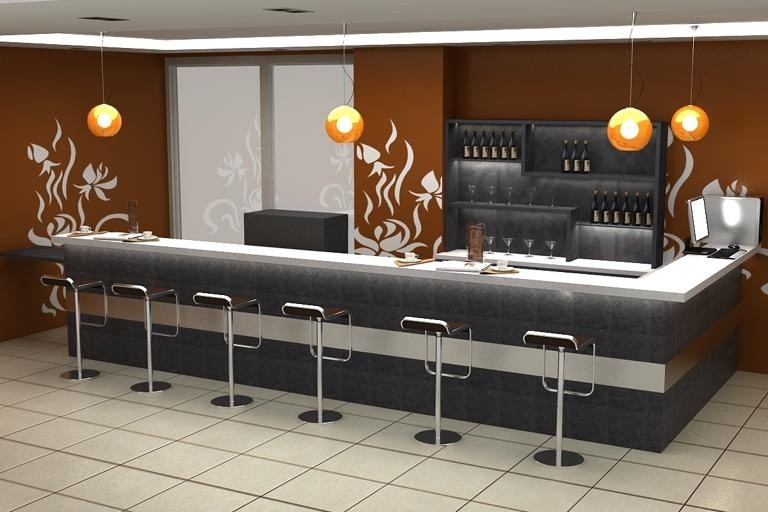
672 25 710 142
607 11 657 149
325 14 364 140
86 29 123 135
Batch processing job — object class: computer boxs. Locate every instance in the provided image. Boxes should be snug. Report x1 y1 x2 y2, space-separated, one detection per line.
702 196 764 247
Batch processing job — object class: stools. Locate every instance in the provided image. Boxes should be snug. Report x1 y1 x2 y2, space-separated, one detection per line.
41 274 108 381
283 303 355 426
113 285 179 394
194 291 285 403
522 327 603 467
401 315 479 443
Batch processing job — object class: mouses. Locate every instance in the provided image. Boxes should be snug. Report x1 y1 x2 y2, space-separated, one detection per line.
728 244 739 248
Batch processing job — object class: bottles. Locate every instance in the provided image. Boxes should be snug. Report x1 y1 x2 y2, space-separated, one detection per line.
633 192 642 225
480 131 488 159
471 131 479 158
561 139 570 173
643 192 653 227
611 191 619 224
621 191 631 225
581 140 592 174
488 132 498 159
571 140 580 172
462 131 469 158
500 131 509 159
591 190 599 224
600 190 610 224
508 132 517 159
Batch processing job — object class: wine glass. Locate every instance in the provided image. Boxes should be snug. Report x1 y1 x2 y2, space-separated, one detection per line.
483 234 496 254
525 184 537 208
502 237 514 256
543 184 559 208
487 185 496 204
468 184 479 204
524 238 535 258
503 186 513 206
546 240 558 259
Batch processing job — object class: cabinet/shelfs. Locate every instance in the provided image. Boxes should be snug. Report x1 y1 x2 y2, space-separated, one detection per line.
242 209 348 254
434 119 669 277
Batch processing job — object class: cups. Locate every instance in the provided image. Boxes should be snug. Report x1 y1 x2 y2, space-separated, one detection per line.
405 252 418 261
80 225 91 232
496 260 508 268
144 231 152 237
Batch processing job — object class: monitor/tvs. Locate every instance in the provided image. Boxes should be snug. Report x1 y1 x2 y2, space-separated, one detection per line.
682 195 717 255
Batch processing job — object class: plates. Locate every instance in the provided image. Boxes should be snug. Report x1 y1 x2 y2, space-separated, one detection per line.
73 231 93 234
400 259 421 263
138 236 158 241
491 267 514 272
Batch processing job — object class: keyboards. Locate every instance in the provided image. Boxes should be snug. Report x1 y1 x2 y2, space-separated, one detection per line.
707 248 739 257
